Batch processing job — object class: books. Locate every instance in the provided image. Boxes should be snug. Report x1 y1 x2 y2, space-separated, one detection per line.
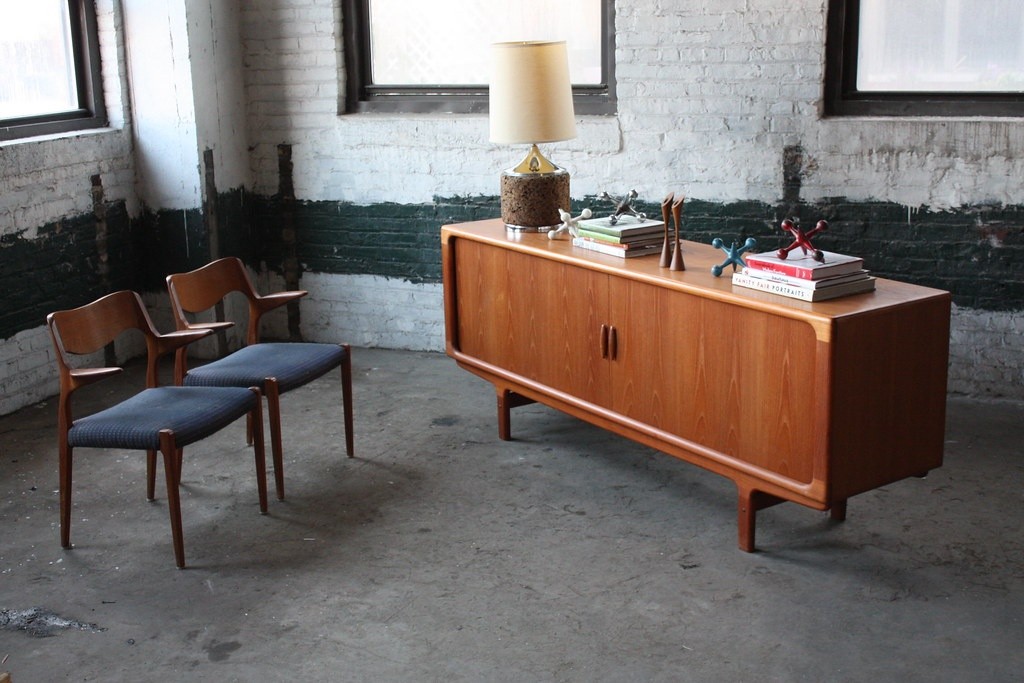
573 214 683 260
732 246 877 302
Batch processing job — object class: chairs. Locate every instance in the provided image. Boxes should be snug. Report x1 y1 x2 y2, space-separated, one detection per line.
47 290 267 569
165 257 354 502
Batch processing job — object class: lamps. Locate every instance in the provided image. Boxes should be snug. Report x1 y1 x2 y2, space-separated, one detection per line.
488 39 579 231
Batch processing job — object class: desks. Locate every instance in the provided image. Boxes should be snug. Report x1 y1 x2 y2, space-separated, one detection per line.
441 217 952 554
